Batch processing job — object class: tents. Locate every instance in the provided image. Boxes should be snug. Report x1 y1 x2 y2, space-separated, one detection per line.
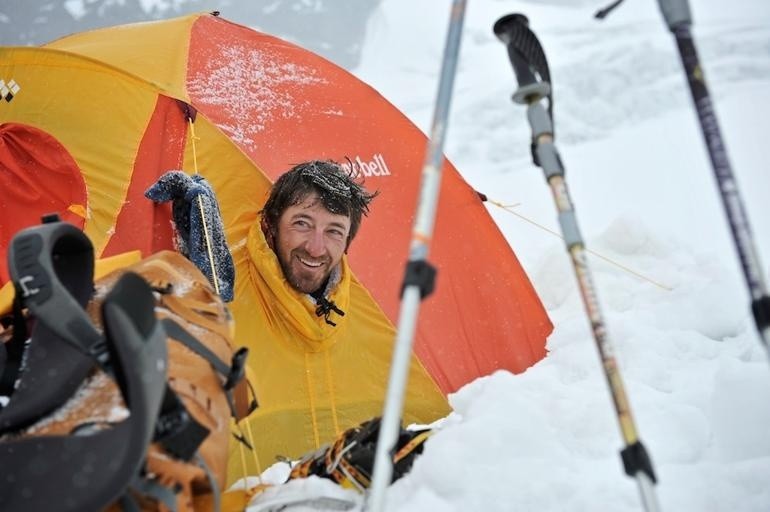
0 12 554 484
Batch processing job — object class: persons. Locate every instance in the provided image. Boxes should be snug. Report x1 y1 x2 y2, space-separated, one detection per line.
256 157 382 296
